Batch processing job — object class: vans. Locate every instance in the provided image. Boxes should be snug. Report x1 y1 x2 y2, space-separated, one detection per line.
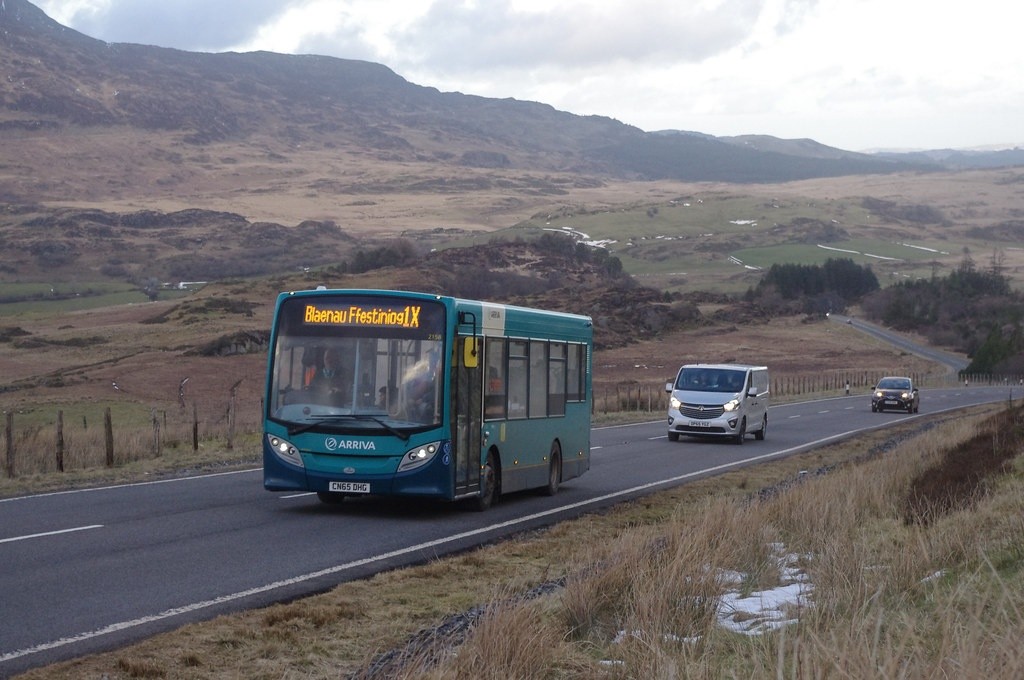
666 363 771 444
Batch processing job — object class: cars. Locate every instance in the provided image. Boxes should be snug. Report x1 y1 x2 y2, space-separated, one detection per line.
871 377 921 416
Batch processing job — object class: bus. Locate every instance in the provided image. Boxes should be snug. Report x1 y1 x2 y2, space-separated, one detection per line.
259 284 595 508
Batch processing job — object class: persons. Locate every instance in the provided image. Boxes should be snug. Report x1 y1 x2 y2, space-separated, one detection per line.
308 348 349 407
376 385 392 410
402 348 442 419
694 371 711 388
